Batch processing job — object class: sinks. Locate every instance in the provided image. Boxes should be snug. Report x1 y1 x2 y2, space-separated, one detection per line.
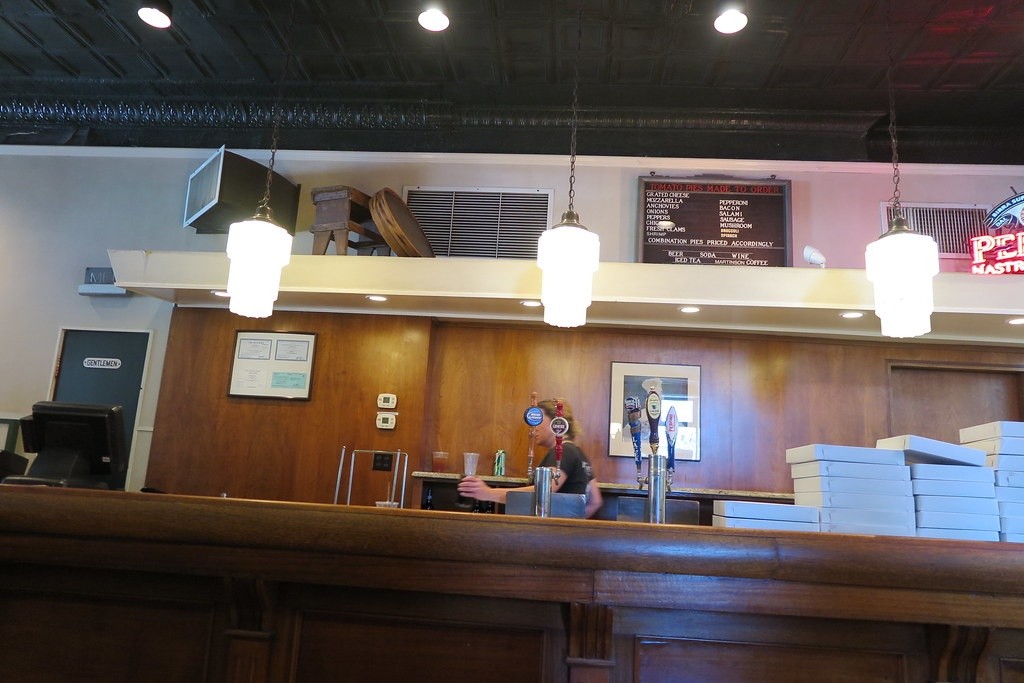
506 491 586 520
617 496 699 526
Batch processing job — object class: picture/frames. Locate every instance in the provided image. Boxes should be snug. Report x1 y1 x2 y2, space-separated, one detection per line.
607 360 702 462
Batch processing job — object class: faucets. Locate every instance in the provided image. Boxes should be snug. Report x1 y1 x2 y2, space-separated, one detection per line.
637 477 644 490
526 470 534 485
666 481 673 492
552 469 561 485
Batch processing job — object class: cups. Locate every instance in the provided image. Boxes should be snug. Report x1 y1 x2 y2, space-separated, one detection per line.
463 452 480 476
432 452 448 472
375 501 399 508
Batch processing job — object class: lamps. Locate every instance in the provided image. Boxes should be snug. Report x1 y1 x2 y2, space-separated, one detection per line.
224 53 295 319
137 0 172 28
803 246 827 268
536 11 601 328
865 26 940 342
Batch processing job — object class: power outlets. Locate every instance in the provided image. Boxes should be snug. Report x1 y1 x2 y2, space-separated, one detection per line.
372 453 394 471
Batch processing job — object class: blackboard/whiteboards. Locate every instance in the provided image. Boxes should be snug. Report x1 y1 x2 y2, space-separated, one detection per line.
634 175 793 267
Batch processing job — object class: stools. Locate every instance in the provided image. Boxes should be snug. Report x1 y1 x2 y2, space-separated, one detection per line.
310 185 392 256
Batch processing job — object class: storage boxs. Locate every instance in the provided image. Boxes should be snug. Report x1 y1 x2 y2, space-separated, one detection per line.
712 421 1024 543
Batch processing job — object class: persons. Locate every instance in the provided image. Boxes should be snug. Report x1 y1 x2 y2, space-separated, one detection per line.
458 398 602 519
